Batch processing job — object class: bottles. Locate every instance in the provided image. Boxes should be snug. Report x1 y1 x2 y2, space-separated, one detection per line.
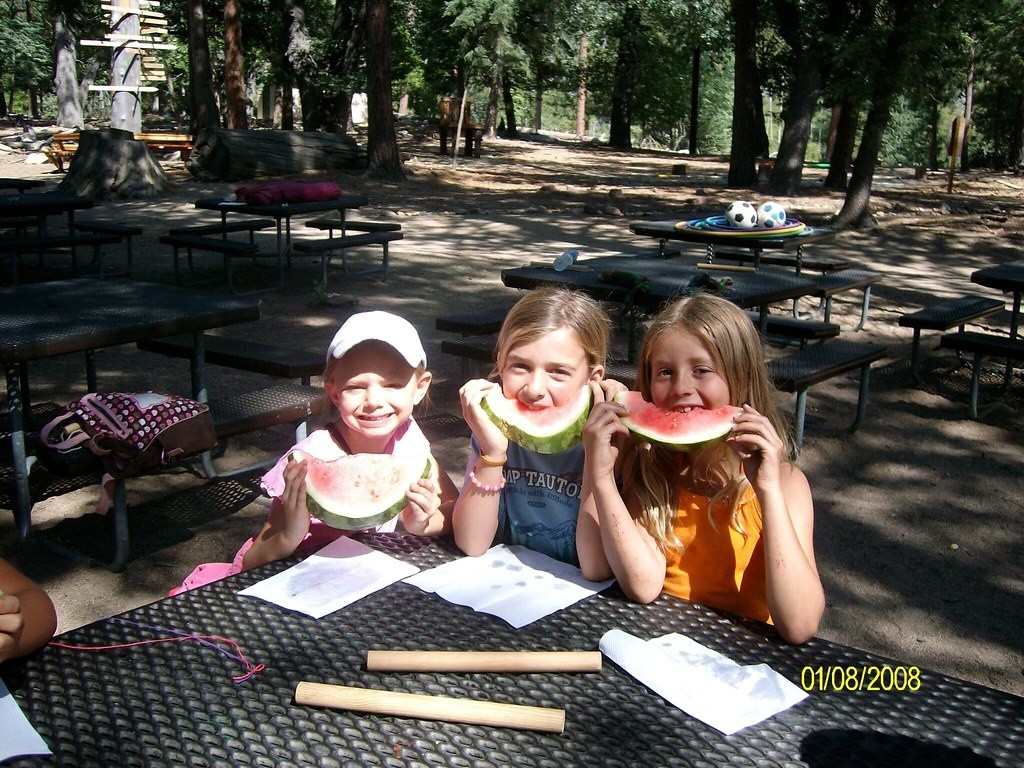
552 251 581 271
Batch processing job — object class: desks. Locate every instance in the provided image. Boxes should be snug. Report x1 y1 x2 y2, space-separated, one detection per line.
629 215 871 348
0 177 134 286
0 275 310 573
173 195 390 298
0 535 1024 768
460 257 869 458
912 259 1024 420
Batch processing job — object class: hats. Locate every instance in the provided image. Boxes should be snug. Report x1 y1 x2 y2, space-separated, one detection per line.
325 311 428 371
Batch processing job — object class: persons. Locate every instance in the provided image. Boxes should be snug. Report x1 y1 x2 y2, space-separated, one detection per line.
272 97 283 130
169 308 459 600
244 98 257 126
141 92 186 124
0 552 57 665
447 287 637 582
583 292 826 644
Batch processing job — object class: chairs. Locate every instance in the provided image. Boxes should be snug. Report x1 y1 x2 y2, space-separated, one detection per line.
439 96 484 158
0 195 1024 473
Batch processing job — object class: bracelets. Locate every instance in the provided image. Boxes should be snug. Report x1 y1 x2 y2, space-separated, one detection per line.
469 466 506 491
480 455 508 466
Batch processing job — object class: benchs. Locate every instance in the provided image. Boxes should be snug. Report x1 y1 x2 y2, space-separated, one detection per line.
45 133 194 173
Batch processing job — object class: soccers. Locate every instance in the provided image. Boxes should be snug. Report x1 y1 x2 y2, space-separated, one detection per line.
725 200 758 228
757 201 787 228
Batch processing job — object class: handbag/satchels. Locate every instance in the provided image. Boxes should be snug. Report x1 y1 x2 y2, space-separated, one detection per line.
42 391 217 468
